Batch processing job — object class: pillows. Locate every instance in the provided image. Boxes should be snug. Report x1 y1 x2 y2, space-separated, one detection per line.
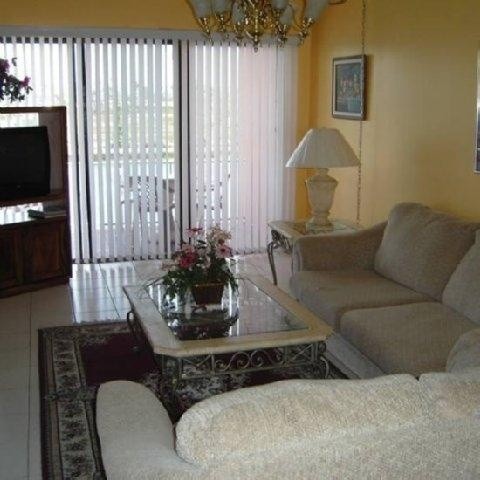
441 230 480 325
373 202 480 301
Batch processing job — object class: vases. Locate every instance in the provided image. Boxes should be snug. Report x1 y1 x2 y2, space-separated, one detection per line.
191 284 224 306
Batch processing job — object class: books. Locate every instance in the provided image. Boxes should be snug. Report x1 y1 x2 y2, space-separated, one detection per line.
28 206 66 218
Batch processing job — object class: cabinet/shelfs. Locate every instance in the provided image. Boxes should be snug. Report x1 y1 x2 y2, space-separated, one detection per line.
0 106 73 299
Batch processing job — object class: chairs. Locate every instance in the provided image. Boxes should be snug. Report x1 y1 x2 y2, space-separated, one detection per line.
120 176 176 253
197 173 232 224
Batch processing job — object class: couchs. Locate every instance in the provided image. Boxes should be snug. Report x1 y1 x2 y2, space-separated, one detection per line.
287 202 480 380
95 330 480 479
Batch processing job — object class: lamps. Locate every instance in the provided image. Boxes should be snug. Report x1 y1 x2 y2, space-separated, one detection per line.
285 128 361 232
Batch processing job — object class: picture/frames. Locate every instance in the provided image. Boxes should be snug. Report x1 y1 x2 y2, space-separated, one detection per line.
331 55 367 121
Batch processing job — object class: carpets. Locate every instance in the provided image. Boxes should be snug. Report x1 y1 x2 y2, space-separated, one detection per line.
38 318 349 479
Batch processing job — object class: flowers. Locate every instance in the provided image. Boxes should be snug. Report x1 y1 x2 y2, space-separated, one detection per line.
0 57 33 103
140 224 239 304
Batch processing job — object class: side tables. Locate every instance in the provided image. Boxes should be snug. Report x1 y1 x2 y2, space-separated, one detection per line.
265 219 366 286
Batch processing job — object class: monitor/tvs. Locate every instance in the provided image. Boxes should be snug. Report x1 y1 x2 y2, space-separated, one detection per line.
0 126 51 207
